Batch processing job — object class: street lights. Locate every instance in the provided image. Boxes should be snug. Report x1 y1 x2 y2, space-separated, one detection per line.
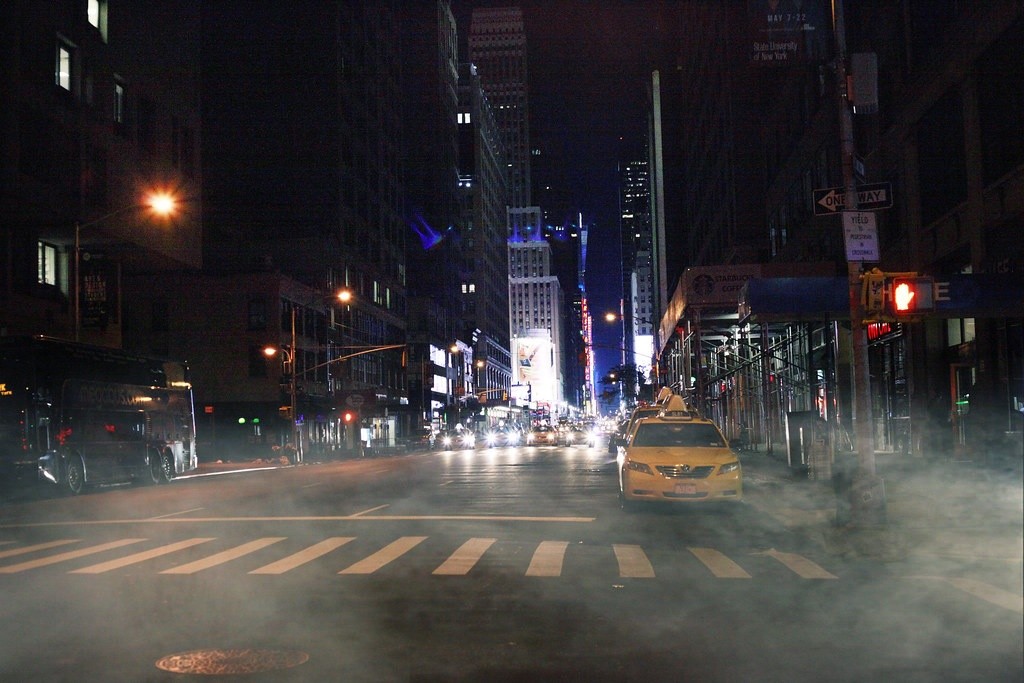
74 194 173 340
264 293 349 464
422 345 459 428
606 314 653 324
455 361 483 422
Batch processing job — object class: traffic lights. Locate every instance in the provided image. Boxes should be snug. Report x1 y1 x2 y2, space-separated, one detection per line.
579 353 587 367
893 278 935 315
503 392 507 401
345 413 352 422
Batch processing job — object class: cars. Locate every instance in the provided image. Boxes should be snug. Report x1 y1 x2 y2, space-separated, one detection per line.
616 387 699 439
406 428 435 450
445 423 475 451
527 420 559 445
615 395 743 513
488 421 519 449
434 432 452 451
565 423 595 447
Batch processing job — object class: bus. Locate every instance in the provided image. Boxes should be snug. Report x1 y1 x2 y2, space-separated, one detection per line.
0 334 198 501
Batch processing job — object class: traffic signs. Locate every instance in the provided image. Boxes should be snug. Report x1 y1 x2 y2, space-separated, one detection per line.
811 182 893 215
